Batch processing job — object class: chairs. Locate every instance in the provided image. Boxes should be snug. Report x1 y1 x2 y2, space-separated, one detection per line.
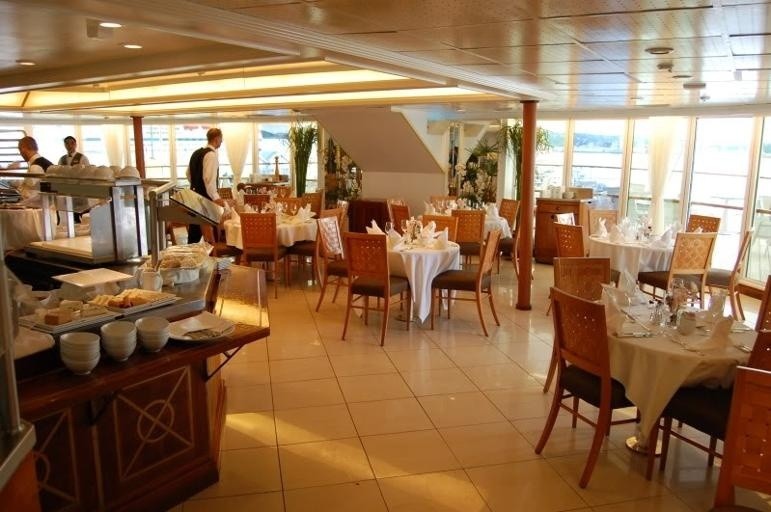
341 230 411 348
533 284 658 491
686 214 722 269
202 175 348 299
554 211 576 229
315 216 351 311
431 226 504 338
388 193 520 279
639 231 719 311
658 326 770 476
587 209 621 236
545 221 585 316
542 255 613 394
699 225 758 323
711 363 770 510
755 273 769 332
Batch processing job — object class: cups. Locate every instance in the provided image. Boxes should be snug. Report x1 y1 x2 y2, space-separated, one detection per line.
139 269 164 293
385 221 393 234
272 186 287 199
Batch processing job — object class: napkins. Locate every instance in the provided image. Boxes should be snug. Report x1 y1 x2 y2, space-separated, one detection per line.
601 289 625 332
618 269 647 306
707 289 727 320
365 219 386 235
660 228 672 248
595 215 608 237
711 315 734 348
608 222 624 244
625 223 638 243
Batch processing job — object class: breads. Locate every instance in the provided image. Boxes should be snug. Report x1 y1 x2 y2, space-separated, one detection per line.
44 287 148 325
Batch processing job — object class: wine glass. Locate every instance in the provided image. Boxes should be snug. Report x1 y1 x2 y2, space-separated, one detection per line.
251 200 298 224
402 217 424 245
624 279 640 320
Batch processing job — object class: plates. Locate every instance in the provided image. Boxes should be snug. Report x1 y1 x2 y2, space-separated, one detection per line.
170 312 238 343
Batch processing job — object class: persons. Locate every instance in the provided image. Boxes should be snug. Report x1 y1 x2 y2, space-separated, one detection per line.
18 136 57 193
185 128 225 244
58 136 90 166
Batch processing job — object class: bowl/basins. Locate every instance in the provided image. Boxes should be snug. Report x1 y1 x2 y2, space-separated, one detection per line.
45 163 141 181
59 316 169 376
